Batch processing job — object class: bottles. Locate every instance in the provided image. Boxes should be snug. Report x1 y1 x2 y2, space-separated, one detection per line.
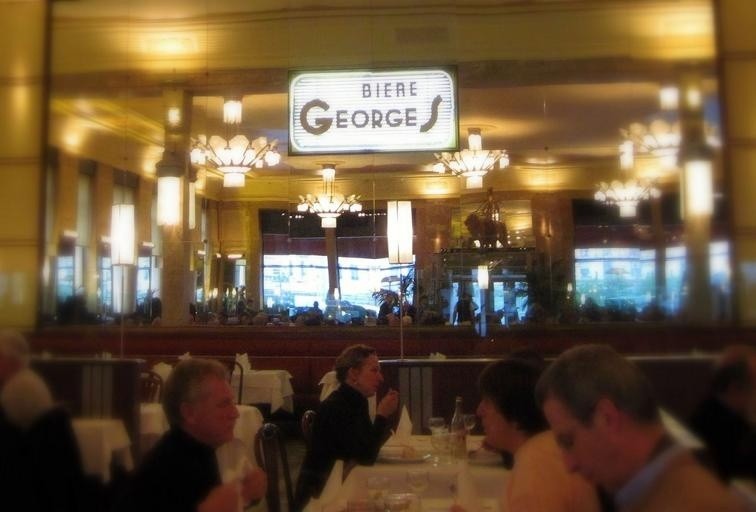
446 394 469 461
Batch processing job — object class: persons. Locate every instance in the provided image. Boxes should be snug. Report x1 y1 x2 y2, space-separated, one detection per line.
480 348 548 471
689 343 756 484
126 312 160 325
294 344 400 512
191 292 546 325
0 327 53 424
131 356 267 511
533 342 745 511
449 358 601 512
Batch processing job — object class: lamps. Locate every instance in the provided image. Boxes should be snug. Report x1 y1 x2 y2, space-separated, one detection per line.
433 128 507 190
477 265 489 290
189 93 281 188
677 117 718 219
296 164 363 229
386 200 413 264
593 139 663 218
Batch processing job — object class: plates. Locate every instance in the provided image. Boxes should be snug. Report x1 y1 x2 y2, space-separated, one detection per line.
381 451 432 466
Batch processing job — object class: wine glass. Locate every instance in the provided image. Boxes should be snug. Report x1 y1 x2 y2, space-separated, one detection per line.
427 414 484 511
346 467 432 512
